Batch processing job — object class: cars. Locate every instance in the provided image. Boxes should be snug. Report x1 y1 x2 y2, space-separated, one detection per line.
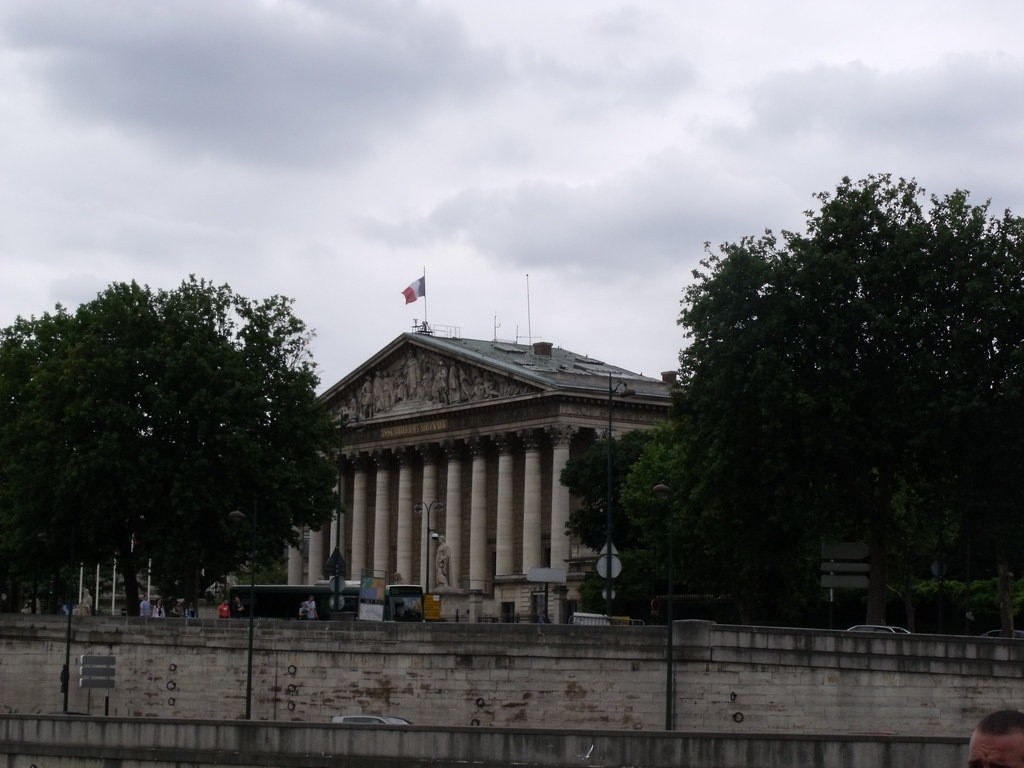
334 715 413 726
846 625 911 634
980 629 1024 639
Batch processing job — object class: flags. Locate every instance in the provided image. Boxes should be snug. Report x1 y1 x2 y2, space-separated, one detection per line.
401 277 425 305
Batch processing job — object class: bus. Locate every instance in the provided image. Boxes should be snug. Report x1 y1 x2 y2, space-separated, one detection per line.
228 584 359 622
314 579 424 624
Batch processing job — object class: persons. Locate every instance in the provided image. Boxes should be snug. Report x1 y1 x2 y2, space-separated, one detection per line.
567 610 576 625
140 594 152 617
228 597 245 619
967 709 1024 768
298 595 319 621
515 612 521 623
152 598 166 618
217 600 231 618
76 588 93 607
184 602 198 619
537 610 551 624
435 535 451 587
397 600 415 617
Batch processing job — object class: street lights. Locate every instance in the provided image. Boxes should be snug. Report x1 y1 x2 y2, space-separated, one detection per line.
606 382 636 617
651 470 676 730
230 508 256 719
413 500 446 595
329 406 367 622
38 516 76 713
